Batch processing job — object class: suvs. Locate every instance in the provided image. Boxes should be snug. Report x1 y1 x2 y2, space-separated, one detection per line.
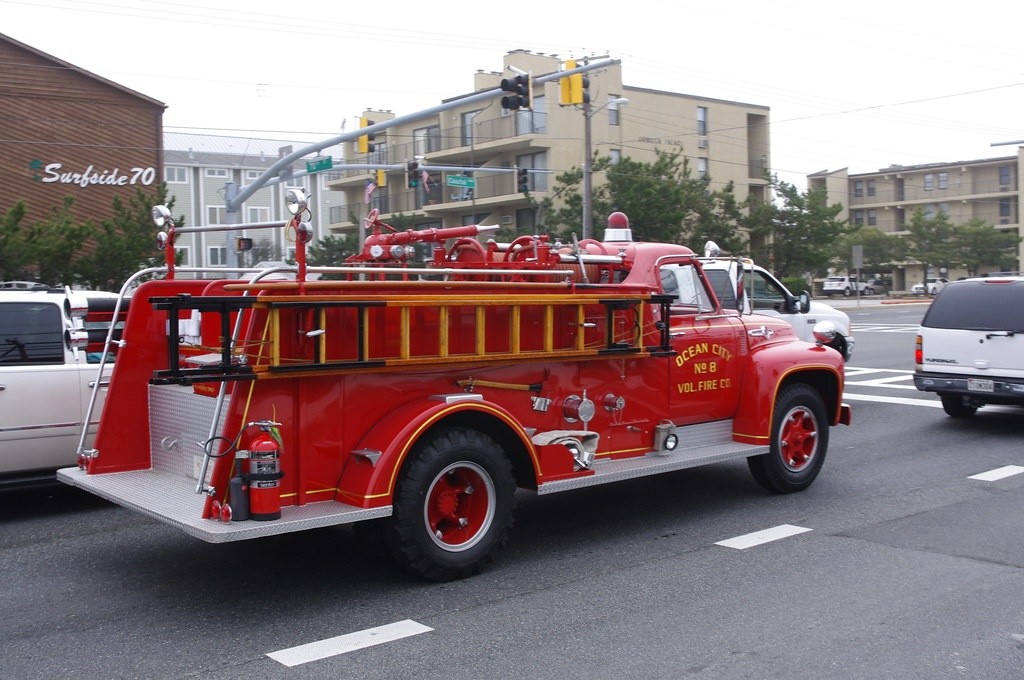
822 276 867 296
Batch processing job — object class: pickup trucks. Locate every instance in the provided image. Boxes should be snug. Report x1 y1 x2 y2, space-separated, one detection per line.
654 240 854 364
0 280 133 493
914 275 1024 416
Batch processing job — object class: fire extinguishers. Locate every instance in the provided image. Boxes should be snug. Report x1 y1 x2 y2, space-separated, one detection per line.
204 418 284 522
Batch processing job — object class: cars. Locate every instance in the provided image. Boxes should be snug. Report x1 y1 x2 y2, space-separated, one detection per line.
866 279 892 295
912 278 949 295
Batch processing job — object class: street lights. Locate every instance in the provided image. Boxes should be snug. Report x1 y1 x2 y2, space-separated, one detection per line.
582 98 630 241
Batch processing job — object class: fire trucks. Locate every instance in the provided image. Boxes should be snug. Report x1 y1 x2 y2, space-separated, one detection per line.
56 188 852 583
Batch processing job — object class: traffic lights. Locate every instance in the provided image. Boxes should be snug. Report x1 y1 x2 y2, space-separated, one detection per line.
515 74 529 107
501 78 518 111
513 166 527 194
238 238 253 251
559 60 590 106
359 117 376 153
405 159 418 190
